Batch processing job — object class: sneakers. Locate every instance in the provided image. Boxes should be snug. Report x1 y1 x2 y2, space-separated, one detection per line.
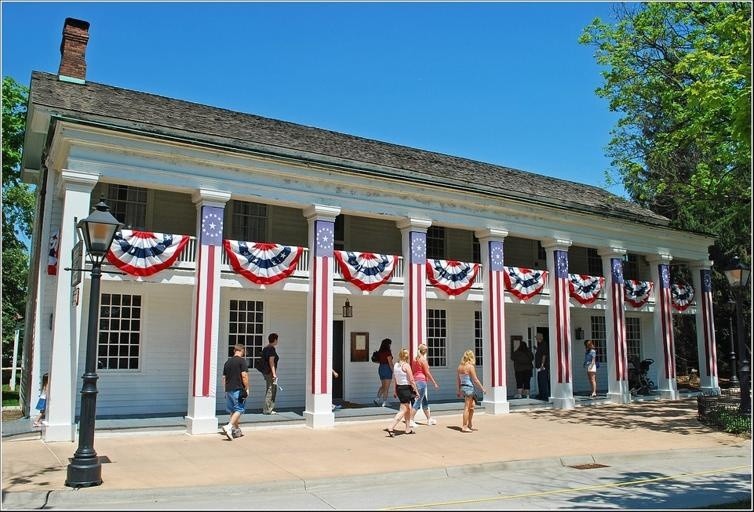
462 427 472 432
222 424 233 440
410 420 418 427
381 401 392 407
426 418 437 425
271 410 276 414
232 427 241 437
373 399 380 406
514 394 522 399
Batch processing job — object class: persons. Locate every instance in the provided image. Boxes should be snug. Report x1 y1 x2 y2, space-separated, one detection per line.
533 333 549 400
33 373 48 426
382 348 420 438
511 341 534 398
457 350 488 431
584 340 597 397
332 369 339 410
262 333 279 415
222 344 249 440
409 344 439 427
374 338 395 407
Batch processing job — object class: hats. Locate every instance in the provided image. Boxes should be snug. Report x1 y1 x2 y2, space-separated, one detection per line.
238 390 247 404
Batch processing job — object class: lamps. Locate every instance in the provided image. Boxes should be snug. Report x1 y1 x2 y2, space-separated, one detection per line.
343 298 352 317
575 328 584 340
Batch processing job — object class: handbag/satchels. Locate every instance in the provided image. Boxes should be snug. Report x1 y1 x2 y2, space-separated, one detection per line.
256 358 270 374
371 350 379 363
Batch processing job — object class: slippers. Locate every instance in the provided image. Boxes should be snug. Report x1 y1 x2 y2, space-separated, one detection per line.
405 429 416 434
386 429 395 437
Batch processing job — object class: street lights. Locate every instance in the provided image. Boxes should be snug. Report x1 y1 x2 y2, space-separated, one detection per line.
721 250 753 417
60 195 130 490
